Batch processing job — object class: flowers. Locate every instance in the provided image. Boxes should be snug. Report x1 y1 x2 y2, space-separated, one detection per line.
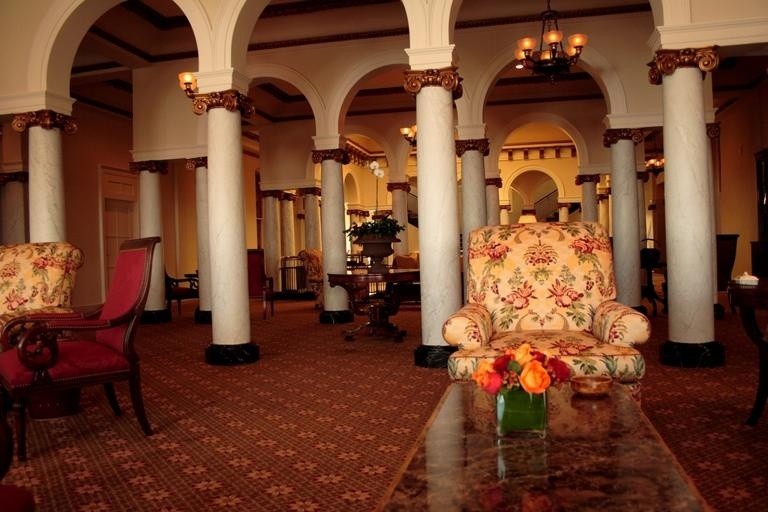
469 340 576 398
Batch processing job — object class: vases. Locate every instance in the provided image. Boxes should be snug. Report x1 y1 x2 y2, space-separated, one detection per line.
496 386 548 439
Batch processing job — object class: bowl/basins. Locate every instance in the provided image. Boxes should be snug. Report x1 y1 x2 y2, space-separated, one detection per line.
571 375 613 395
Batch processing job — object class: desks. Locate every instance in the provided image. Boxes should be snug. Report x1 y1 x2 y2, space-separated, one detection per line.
183 273 199 278
324 267 421 345
367 375 714 510
722 277 767 429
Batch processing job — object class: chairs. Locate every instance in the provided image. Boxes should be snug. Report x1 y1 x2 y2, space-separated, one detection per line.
297 245 370 312
247 248 277 321
661 230 741 314
0 235 165 464
162 260 201 317
436 219 653 414
638 247 667 316
0 240 86 343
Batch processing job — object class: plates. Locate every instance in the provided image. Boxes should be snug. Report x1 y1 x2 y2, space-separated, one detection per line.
734 279 759 285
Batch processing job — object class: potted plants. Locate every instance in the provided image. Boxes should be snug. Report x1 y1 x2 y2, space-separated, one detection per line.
340 208 407 275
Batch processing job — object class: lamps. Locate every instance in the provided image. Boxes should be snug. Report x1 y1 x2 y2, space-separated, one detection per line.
177 70 198 101
399 124 418 148
510 1 592 85
